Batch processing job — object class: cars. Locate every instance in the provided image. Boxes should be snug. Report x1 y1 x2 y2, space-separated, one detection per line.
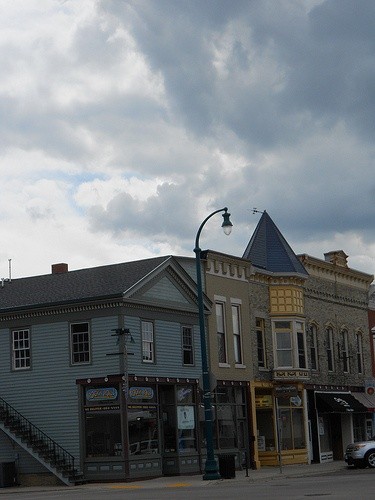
345 435 375 468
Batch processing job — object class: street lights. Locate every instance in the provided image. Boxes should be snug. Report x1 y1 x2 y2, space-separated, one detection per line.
193 207 232 480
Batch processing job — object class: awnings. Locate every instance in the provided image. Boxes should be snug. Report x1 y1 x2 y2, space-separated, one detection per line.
315 391 375 415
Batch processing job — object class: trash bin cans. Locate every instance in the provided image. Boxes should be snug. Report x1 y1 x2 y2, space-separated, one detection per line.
216 450 236 480
